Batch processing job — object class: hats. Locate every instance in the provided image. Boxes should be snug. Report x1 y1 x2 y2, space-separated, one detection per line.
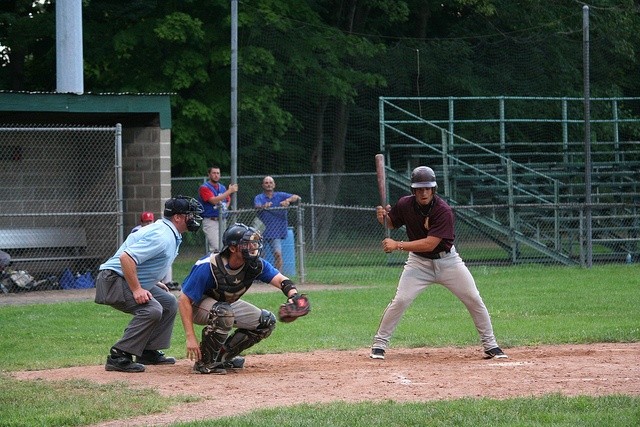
141 212 156 222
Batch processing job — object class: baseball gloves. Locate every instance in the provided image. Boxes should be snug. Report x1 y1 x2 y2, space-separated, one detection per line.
278 293 310 323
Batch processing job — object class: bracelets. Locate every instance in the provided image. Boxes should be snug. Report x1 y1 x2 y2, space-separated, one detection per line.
398 242 400 250
400 241 404 252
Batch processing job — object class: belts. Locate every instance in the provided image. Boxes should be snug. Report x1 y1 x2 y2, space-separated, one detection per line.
414 251 451 259
210 217 219 221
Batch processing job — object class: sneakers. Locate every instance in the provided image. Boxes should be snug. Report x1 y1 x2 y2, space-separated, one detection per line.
193 362 227 375
221 357 245 370
482 348 508 359
105 355 145 373
370 348 385 359
136 350 176 364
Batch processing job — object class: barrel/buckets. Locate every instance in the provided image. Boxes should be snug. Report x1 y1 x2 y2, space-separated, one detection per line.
261 227 296 276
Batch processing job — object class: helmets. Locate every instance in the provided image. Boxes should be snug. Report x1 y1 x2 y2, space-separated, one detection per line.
410 166 438 195
163 195 205 231
219 223 264 270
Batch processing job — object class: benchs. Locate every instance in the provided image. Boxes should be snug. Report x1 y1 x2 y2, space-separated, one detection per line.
0 227 99 276
383 140 640 259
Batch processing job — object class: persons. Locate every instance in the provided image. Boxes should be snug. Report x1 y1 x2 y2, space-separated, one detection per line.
131 212 154 233
196 167 238 253
94 194 204 372
254 176 299 273
369 166 507 360
178 222 310 375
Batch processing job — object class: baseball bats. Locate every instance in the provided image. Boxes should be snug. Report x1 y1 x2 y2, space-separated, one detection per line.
375 153 392 253
